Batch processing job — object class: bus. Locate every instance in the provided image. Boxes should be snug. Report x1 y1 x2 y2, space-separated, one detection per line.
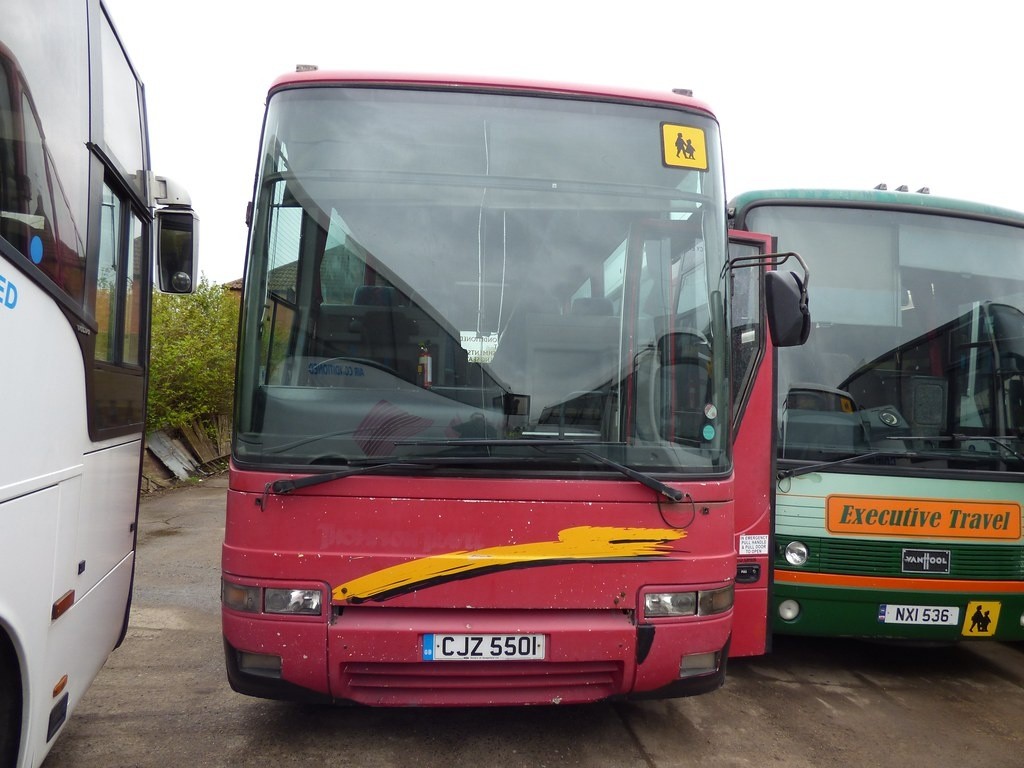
0 2 199 768
730 184 1024 656
218 62 812 716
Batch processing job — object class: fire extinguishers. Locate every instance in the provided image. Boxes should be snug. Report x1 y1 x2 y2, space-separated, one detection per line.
417 342 433 389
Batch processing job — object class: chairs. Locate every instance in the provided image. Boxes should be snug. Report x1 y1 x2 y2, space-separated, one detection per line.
307 286 620 388
